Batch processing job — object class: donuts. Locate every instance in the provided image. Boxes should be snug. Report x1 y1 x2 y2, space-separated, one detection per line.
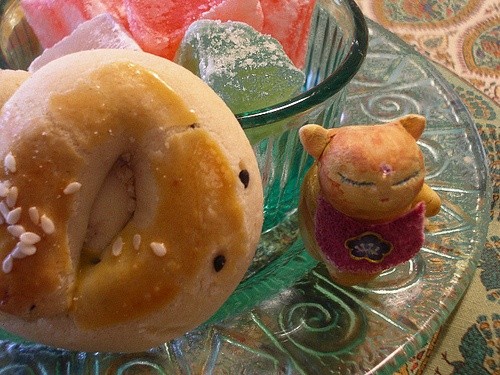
1 47 263 353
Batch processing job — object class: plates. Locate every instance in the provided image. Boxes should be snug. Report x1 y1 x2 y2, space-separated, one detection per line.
0 16 492 373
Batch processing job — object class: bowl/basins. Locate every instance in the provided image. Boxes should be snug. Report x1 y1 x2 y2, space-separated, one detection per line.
0 0 367 238
